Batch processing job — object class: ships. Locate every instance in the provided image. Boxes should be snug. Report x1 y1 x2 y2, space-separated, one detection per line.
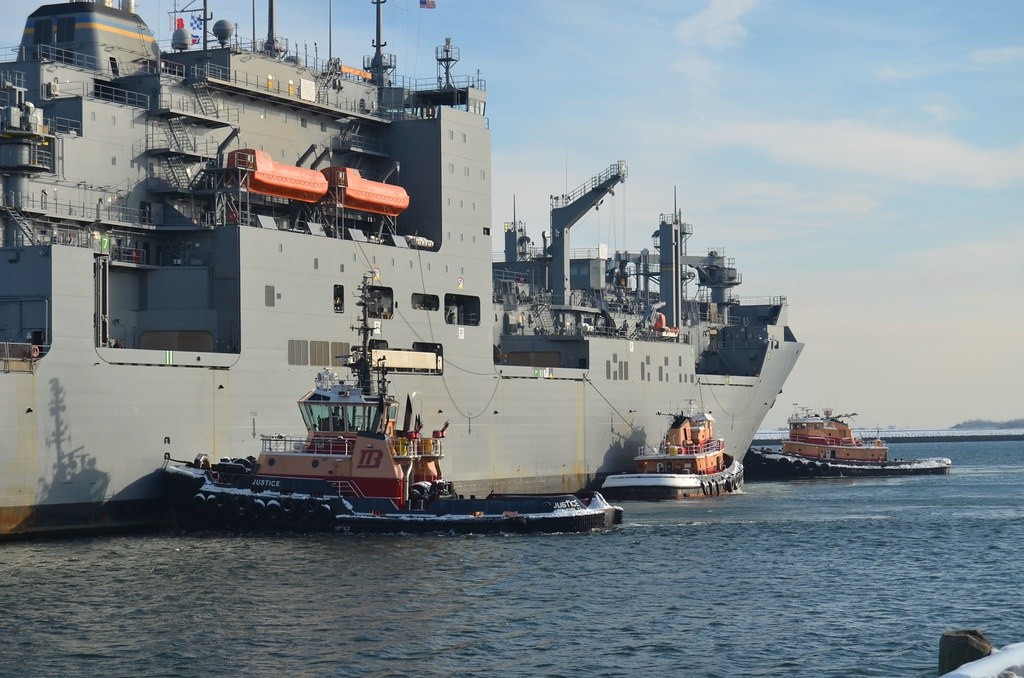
0 0 803 541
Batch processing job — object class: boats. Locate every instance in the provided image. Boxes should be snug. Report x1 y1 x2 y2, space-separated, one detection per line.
746 403 951 479
162 255 624 537
601 398 743 501
